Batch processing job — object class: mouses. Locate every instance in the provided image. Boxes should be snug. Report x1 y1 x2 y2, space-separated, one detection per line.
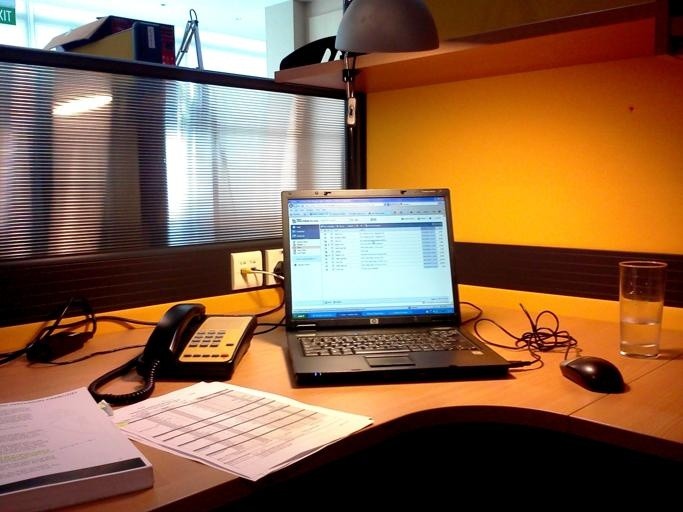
560 355 625 395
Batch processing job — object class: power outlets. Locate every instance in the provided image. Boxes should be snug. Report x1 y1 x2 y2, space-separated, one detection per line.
265 249 285 286
230 251 265 290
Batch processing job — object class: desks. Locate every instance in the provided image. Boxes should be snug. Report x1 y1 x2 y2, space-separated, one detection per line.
1 307 682 511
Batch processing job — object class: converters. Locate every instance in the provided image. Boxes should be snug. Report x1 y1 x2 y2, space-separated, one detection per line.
26 330 95 364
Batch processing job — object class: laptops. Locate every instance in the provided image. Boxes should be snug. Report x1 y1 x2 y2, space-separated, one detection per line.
280 187 513 388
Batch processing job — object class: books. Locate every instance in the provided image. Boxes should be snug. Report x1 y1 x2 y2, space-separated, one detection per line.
0 386 155 511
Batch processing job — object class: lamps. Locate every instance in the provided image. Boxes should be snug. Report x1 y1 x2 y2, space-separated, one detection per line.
335 0 440 189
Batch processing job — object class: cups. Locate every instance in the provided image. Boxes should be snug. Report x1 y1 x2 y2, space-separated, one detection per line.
620 260 667 357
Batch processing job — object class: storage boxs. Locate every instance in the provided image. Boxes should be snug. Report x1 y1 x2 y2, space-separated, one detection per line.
44 16 175 64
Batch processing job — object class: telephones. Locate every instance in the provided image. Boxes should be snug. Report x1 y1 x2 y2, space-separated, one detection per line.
137 303 257 381
